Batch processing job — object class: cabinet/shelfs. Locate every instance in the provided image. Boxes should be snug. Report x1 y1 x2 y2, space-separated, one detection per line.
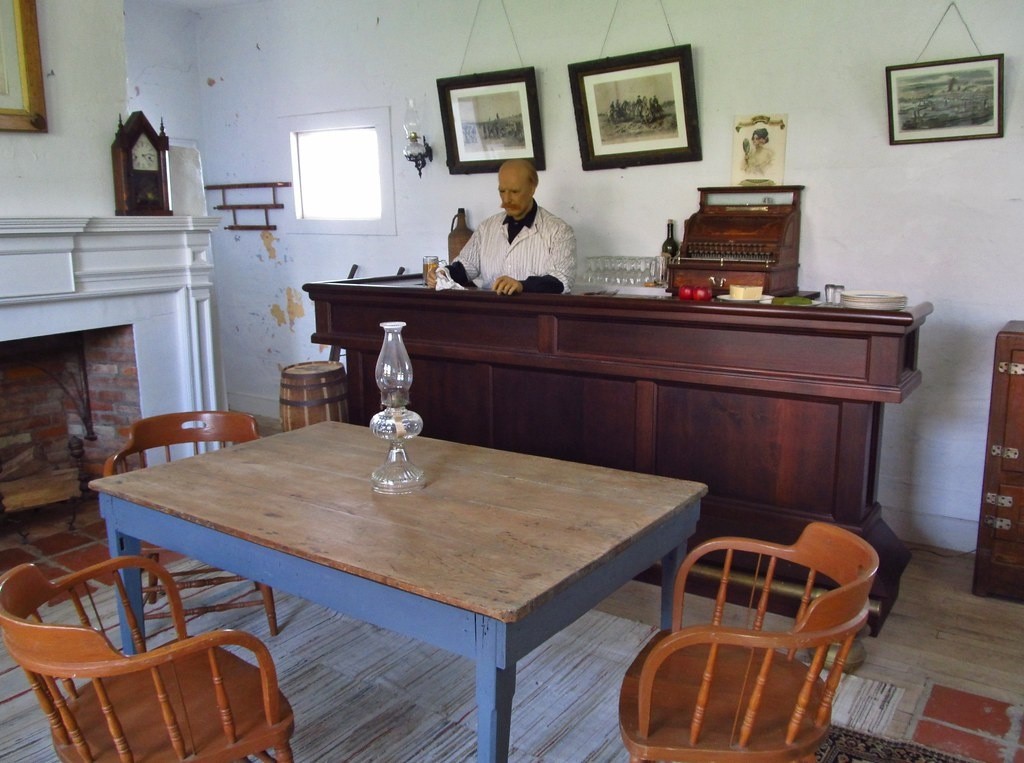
971 319 1024 605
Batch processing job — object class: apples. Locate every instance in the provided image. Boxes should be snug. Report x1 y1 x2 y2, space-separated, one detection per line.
679 285 712 301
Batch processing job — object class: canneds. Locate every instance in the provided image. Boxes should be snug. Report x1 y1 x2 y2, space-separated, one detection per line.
423 256 438 283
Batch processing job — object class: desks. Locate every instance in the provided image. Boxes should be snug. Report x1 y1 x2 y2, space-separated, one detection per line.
88 420 708 763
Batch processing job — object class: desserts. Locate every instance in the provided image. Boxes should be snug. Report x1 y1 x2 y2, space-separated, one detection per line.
729 284 763 299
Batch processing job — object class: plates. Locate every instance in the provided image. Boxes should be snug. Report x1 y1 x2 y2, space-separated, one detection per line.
717 294 774 301
758 298 823 307
841 290 907 311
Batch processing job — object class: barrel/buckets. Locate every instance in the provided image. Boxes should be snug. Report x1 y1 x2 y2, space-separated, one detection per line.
279 361 350 433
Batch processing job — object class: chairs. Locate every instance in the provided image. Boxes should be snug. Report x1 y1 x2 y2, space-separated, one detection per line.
0 555 294 763
103 409 279 637
618 521 879 763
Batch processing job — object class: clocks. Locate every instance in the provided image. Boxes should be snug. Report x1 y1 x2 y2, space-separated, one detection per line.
111 110 173 217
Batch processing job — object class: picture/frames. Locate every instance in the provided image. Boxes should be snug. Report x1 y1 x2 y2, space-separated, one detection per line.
0 0 49 135
885 53 1004 146
567 43 703 172
435 65 546 175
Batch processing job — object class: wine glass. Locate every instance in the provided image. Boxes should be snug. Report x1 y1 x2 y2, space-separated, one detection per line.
581 257 666 286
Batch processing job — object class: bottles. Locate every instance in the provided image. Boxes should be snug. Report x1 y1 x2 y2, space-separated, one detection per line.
834 286 844 302
825 285 834 302
662 219 679 282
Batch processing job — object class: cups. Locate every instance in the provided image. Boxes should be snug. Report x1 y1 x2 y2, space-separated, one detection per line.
422 256 447 285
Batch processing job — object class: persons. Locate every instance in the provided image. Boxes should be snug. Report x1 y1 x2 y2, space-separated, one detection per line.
426 158 578 295
610 94 660 116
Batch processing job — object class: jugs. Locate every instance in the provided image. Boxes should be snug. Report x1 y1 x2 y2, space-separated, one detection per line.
448 207 475 267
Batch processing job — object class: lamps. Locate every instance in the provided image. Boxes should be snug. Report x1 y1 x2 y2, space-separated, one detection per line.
370 323 425 495
403 97 433 179
